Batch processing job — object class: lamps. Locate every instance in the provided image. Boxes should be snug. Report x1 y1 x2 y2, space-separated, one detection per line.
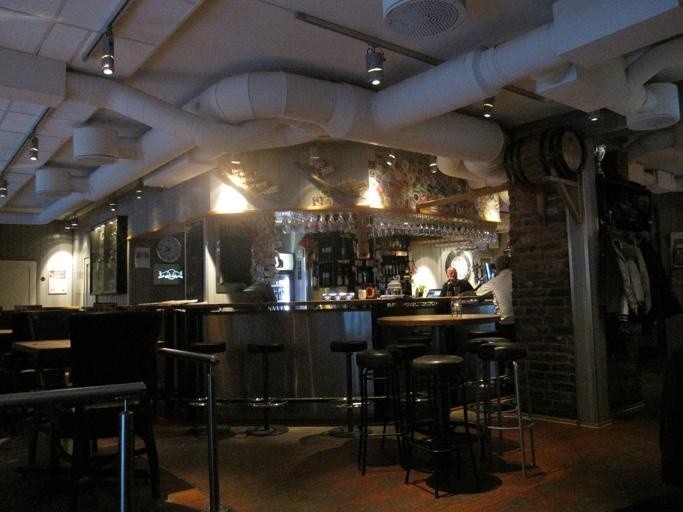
0 132 39 199
98 27 115 78
364 38 497 121
62 176 145 230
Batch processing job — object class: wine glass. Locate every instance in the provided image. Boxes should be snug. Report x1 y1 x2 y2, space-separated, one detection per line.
369 212 496 250
595 145 607 175
278 210 359 235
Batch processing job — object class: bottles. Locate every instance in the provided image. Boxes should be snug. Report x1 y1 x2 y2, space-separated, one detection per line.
446 281 453 296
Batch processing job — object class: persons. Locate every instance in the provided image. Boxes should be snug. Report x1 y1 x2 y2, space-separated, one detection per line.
476 255 512 335
438 267 475 297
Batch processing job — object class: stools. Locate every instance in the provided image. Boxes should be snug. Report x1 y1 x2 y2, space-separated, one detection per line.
329 339 369 438
353 312 540 499
247 342 290 437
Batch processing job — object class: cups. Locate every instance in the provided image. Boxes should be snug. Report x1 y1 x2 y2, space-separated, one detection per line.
357 289 366 300
453 286 460 296
451 298 463 317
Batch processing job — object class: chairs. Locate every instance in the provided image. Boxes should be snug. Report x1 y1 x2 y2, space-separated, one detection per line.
0 301 230 510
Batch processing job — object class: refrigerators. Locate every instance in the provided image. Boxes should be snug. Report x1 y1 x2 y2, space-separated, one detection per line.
267 252 296 310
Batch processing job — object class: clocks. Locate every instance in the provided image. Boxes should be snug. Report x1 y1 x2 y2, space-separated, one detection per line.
156 235 183 264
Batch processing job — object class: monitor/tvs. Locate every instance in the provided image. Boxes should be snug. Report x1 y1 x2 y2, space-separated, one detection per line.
426 289 442 297
